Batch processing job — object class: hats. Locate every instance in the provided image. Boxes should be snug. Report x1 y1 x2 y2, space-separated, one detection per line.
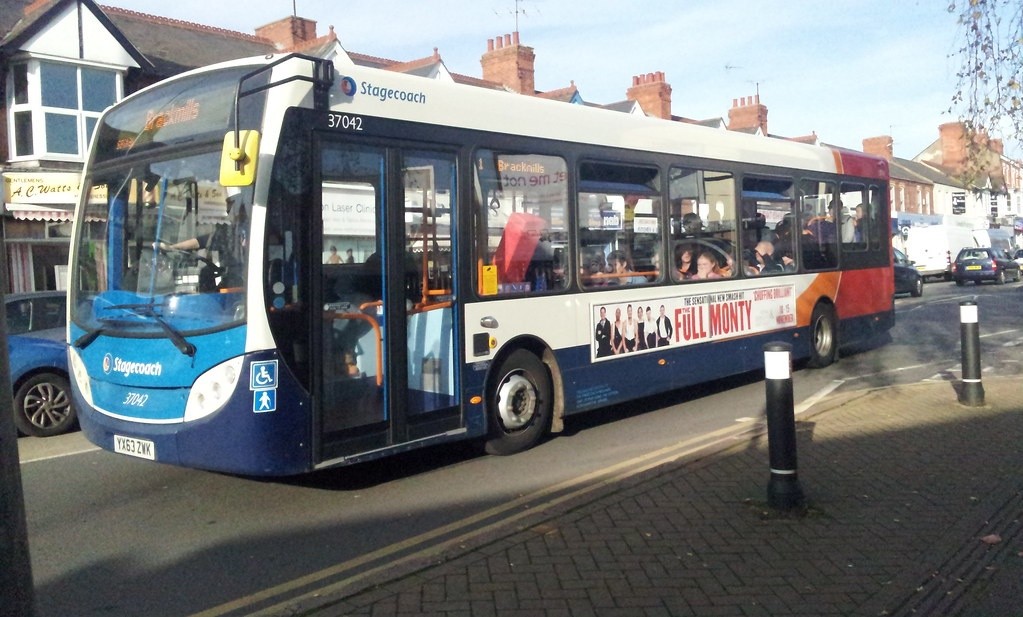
827 199 843 209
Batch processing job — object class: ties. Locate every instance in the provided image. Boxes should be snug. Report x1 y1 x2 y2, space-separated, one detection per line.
601 321 605 327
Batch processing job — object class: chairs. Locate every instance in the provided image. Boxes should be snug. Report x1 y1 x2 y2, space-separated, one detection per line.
807 216 832 226
723 266 758 275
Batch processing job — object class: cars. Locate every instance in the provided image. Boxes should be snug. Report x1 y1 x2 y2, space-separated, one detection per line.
892 247 924 297
1012 249 1023 275
949 247 1021 286
4 289 80 439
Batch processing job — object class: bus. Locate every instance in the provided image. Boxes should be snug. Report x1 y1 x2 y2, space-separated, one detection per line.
62 53 897 480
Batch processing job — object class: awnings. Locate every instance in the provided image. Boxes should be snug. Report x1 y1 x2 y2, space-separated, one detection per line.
411 232 504 254
3 201 234 226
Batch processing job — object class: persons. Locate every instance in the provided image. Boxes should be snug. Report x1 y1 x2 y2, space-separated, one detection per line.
151 194 250 296
671 210 736 285
328 245 355 264
596 304 673 358
581 226 662 289
741 198 883 277
